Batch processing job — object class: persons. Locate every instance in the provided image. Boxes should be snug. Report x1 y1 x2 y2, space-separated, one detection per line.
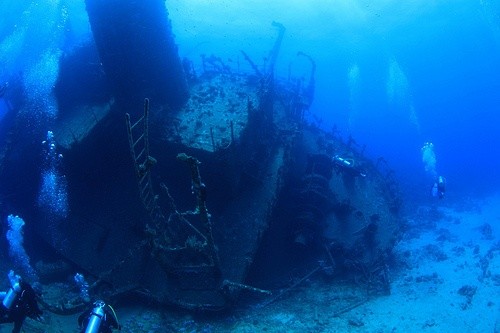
431 176 446 198
78 300 120 333
0 281 38 333
